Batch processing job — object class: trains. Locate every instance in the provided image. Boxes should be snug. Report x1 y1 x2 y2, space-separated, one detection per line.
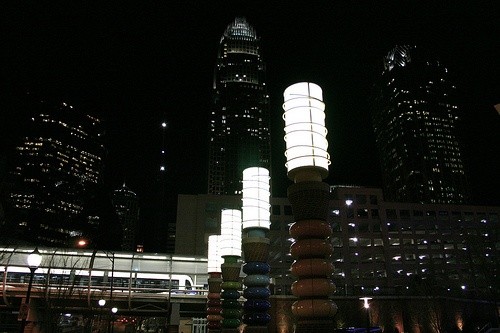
0 265 196 299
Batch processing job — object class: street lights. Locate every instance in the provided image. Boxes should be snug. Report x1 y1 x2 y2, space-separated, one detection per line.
219 206 242 333
111 306 119 333
239 165 272 333
75 239 115 299
19 245 44 333
97 297 106 333
282 83 340 333
207 233 222 333
